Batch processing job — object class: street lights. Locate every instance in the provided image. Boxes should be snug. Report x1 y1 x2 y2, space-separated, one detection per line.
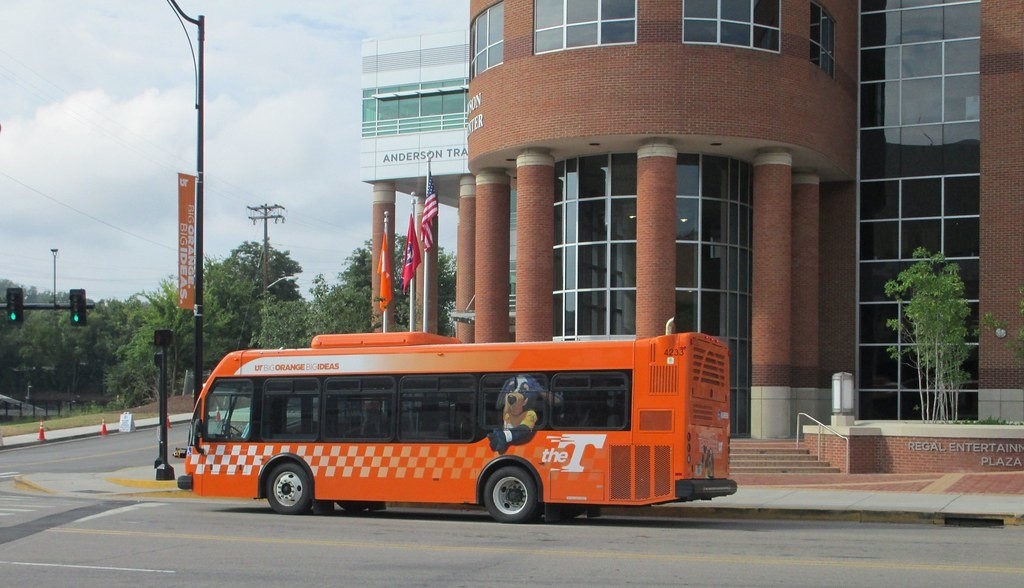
50 247 60 328
261 276 299 348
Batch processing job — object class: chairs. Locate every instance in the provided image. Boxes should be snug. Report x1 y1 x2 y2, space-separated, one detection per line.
574 409 594 427
607 415 620 428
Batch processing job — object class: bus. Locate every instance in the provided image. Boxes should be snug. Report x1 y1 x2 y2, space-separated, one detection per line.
173 317 738 526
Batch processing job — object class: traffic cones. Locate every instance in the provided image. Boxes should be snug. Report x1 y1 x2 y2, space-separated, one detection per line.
36 419 47 440
166 413 172 429
99 417 108 436
215 406 222 422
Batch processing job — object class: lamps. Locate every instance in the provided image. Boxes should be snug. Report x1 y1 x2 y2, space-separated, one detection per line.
832 372 855 416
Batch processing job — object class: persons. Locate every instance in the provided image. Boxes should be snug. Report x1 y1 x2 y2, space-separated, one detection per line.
225 422 249 439
363 399 384 435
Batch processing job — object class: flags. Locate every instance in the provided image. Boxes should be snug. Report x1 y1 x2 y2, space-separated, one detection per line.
376 230 394 312
421 171 438 254
401 213 422 295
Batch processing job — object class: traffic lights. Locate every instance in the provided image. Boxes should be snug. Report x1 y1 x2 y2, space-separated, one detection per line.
69 289 87 328
5 286 25 326
154 329 172 347
153 352 163 367
